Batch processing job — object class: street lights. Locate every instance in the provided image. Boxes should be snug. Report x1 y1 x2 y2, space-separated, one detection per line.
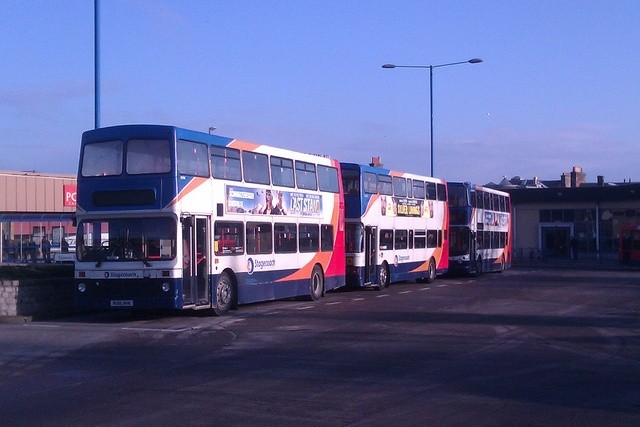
382 58 483 179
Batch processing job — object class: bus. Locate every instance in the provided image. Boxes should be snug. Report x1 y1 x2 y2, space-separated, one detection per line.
339 161 451 292
446 180 514 277
73 123 347 318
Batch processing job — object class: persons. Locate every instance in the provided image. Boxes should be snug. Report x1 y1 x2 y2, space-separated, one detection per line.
42 236 52 263
6 237 38 264
381 196 386 214
569 235 578 260
263 190 275 214
428 201 433 217
274 191 287 215
